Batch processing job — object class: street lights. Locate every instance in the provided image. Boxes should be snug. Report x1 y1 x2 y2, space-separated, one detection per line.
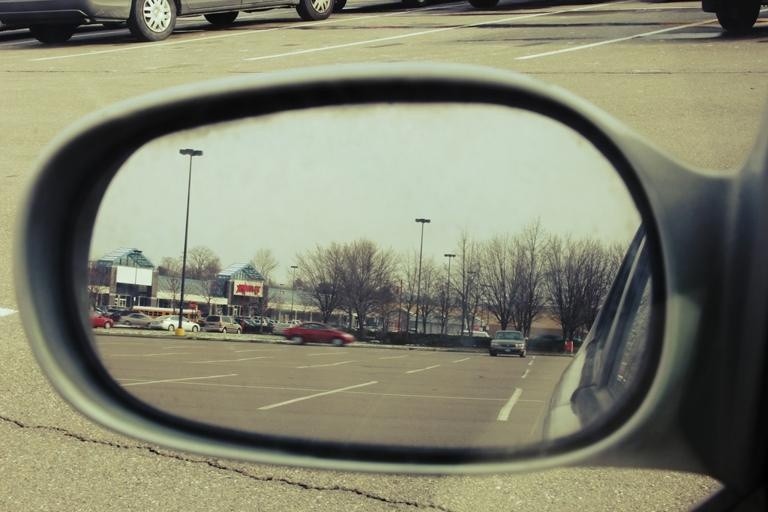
132 247 143 303
414 217 432 344
290 265 298 322
479 283 495 331
444 254 456 343
467 270 476 336
175 148 203 336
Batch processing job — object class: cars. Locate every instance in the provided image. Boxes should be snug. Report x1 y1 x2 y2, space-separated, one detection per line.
283 322 355 346
535 219 651 445
118 312 154 326
235 314 301 336
529 333 584 343
88 305 131 329
1 1 348 45
205 314 242 334
490 330 528 357
151 315 201 333
701 1 767 32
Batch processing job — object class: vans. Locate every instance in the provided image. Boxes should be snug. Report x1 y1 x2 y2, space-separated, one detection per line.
458 330 490 339
